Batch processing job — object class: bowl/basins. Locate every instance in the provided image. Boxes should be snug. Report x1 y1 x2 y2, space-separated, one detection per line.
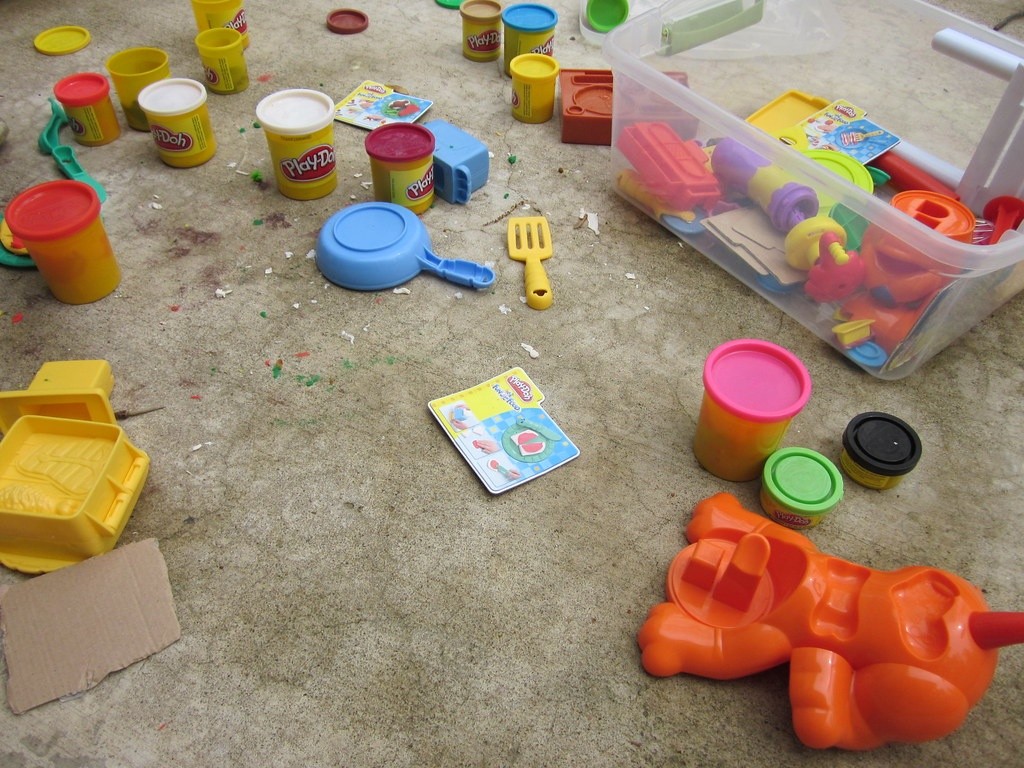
759 447 844 531
840 412 922 490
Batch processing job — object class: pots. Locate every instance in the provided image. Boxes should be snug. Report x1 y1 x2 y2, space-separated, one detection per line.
316 201 496 292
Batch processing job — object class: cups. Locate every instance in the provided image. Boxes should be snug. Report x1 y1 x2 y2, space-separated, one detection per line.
365 122 436 214
195 27 249 94
693 339 812 483
3 179 121 304
53 72 120 146
501 2 559 78
190 0 250 50
138 77 214 169
510 53 559 124
106 47 172 133
459 0 502 62
255 89 336 200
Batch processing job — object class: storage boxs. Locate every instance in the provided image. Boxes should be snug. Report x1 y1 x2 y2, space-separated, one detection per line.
600 0 1024 380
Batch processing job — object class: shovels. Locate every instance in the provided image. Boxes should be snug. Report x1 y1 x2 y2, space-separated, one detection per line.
507 216 554 310
52 145 107 205
38 98 69 154
